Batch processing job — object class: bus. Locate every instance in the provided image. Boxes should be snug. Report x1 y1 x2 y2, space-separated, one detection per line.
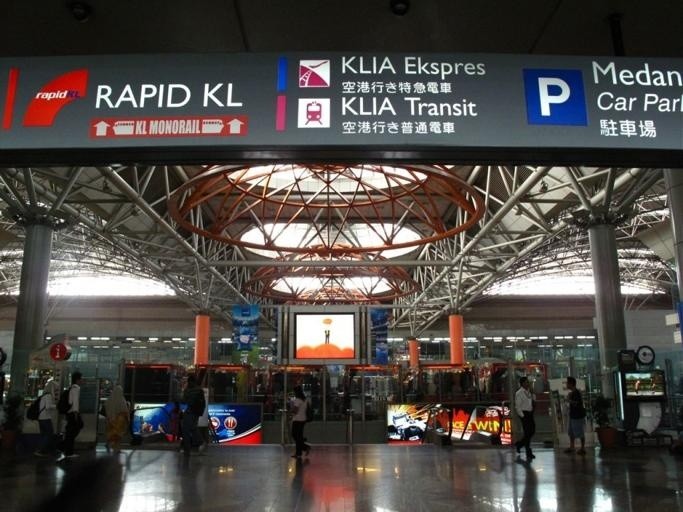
121 359 550 419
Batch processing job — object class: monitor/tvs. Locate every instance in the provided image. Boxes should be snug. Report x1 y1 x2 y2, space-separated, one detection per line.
288 305 361 365
624 370 664 398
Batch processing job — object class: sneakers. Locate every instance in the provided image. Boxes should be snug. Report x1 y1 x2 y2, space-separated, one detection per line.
291 445 311 458
563 448 586 455
176 444 204 456
33 449 80 461
515 441 536 458
103 443 121 454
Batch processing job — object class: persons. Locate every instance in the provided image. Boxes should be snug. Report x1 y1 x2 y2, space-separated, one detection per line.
292 456 309 512
181 373 205 456
288 386 311 457
515 455 540 512
180 455 205 512
63 372 83 458
564 376 586 455
104 385 131 451
34 380 59 457
568 453 593 512
514 377 536 458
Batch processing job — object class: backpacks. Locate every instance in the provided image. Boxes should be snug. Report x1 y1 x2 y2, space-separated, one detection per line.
57 385 77 415
26 392 53 420
